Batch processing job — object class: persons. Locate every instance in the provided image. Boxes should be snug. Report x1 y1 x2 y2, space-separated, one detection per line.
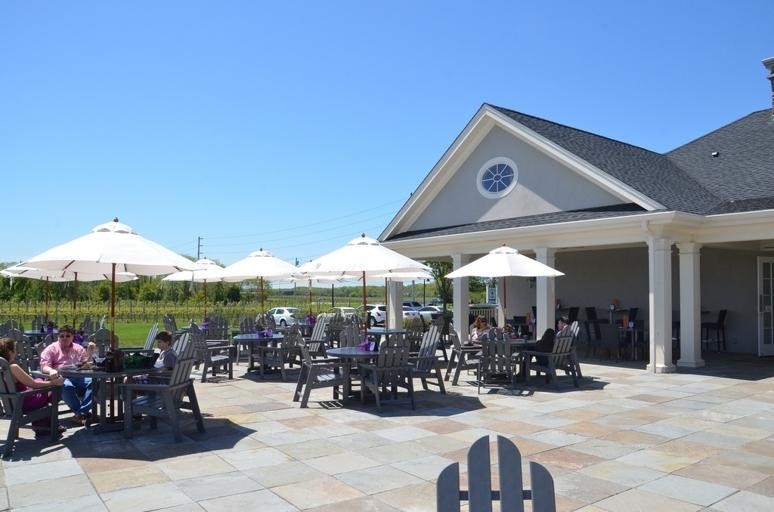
154 331 177 368
471 315 493 345
40 325 94 423
515 316 571 381
0 338 66 432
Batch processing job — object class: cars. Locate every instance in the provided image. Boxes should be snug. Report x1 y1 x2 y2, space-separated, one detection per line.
417 303 452 321
402 300 422 309
255 306 304 328
403 306 420 320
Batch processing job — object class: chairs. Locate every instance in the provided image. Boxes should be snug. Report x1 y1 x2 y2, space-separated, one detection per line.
702 309 728 353
0 307 652 459
433 434 557 511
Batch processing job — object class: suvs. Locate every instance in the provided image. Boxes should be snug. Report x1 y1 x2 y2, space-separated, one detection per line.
317 306 358 324
357 304 387 326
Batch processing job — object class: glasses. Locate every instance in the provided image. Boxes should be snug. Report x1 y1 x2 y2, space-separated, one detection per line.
60 335 70 338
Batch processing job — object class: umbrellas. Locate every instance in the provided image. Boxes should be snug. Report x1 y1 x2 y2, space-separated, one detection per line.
444 243 566 279
